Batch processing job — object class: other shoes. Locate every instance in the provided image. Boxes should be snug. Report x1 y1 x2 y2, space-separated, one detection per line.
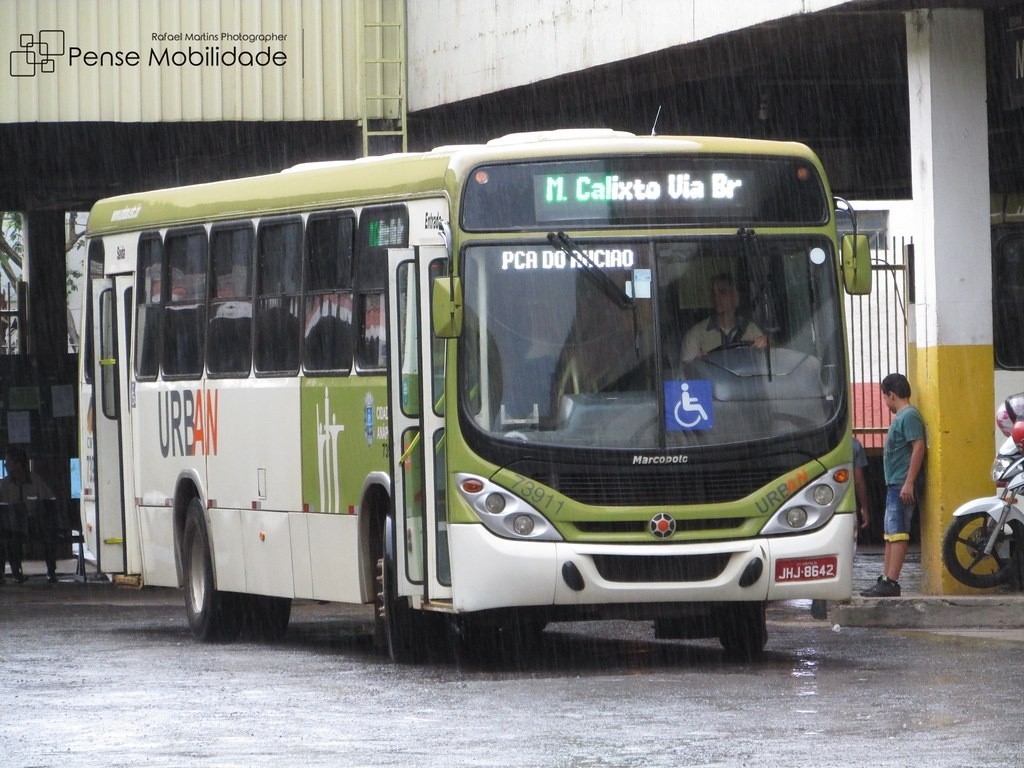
46 570 57 582
11 570 24 583
810 597 827 621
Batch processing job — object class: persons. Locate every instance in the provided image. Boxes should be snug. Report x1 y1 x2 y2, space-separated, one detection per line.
0 446 57 583
860 373 926 597
810 436 869 620
680 272 767 364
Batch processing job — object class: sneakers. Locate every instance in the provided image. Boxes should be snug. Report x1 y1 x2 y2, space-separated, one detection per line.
859 575 902 597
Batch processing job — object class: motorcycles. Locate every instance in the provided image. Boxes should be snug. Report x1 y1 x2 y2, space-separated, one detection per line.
940 403 1024 597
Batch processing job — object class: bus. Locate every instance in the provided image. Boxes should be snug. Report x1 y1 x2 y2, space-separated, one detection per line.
75 126 873 666
